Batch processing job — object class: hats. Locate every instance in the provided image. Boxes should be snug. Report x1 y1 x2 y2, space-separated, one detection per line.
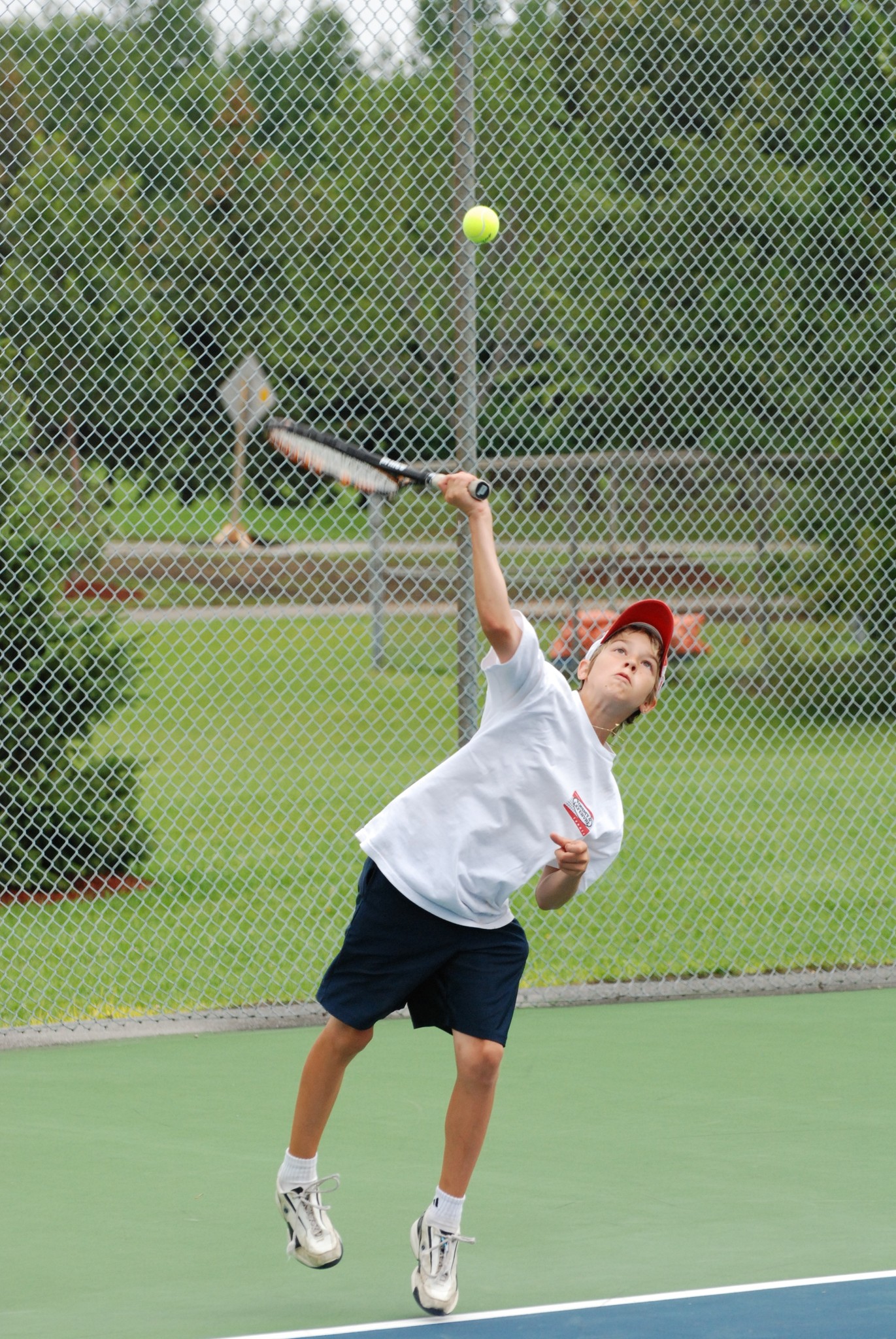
585 599 675 701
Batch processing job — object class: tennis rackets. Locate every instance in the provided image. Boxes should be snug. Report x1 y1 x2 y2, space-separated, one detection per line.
265 418 492 502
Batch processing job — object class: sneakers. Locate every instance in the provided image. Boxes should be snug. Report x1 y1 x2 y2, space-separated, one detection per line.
409 1208 474 1316
275 1175 345 1269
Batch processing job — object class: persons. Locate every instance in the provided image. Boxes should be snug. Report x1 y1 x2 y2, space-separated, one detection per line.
275 471 675 1318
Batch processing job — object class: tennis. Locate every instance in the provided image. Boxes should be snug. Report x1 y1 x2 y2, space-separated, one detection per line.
462 205 501 245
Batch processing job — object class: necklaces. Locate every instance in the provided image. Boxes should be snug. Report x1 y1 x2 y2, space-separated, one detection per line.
592 724 625 742
